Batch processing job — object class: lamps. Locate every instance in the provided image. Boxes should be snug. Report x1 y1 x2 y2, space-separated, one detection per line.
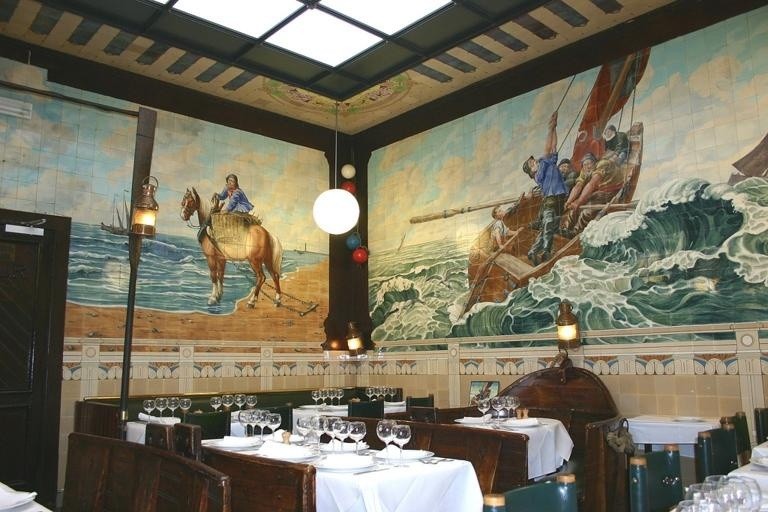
347 321 367 368
126 176 160 236
314 102 362 236
536 299 582 382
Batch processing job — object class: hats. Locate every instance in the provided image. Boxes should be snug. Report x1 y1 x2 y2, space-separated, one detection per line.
226 174 238 184
604 125 616 135
580 153 596 167
523 155 534 179
558 159 570 167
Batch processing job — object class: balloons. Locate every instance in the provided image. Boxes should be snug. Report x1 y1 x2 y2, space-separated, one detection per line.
346 235 368 265
341 164 357 193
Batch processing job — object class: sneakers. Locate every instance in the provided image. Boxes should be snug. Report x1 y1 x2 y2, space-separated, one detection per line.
543 251 551 261
527 251 537 266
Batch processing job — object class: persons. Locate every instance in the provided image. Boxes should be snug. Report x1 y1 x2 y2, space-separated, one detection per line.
489 203 525 255
522 110 571 266
594 123 632 166
207 175 251 218
558 152 625 240
526 156 578 235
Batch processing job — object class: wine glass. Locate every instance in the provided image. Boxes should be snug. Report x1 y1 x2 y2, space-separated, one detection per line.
312 384 397 404
295 415 343 453
332 421 352 453
478 394 520 428
209 393 283 442
391 425 412 466
347 421 367 457
676 473 764 512
142 396 192 424
375 420 398 465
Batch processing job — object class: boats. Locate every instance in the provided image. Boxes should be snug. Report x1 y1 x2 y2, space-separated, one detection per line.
457 121 643 314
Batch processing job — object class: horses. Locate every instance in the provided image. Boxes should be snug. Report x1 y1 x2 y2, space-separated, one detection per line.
180 186 284 308
